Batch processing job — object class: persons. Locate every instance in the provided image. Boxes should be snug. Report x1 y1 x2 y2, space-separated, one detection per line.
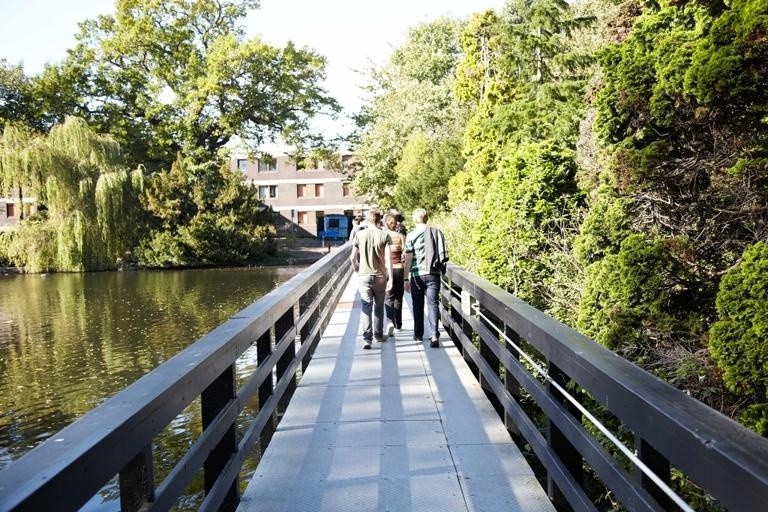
349 207 449 349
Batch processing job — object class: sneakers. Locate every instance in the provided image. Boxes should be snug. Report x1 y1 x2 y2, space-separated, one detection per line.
413 336 439 348
363 322 401 349
